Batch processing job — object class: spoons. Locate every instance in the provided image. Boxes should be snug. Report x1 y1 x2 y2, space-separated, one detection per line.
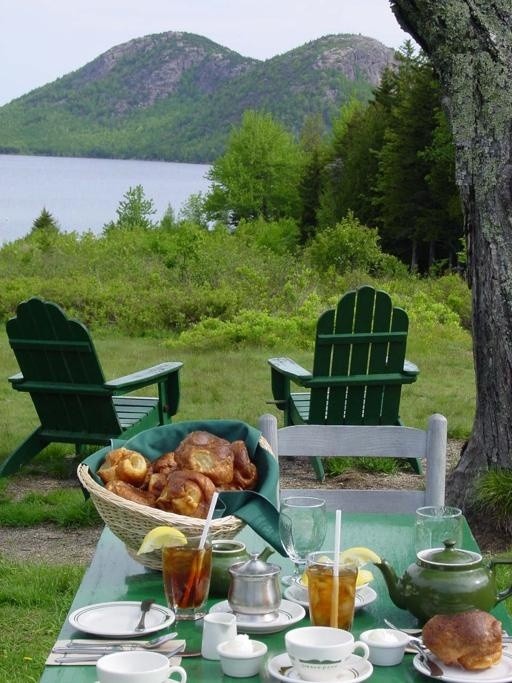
66 631 178 649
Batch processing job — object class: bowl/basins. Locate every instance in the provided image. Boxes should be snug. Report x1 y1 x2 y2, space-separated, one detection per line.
296 577 370 593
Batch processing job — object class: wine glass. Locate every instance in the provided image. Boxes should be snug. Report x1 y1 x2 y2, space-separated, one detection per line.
415 505 464 553
278 496 328 585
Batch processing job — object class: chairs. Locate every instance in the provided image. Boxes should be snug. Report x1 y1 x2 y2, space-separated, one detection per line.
265 284 422 482
0 296 184 479
257 413 448 513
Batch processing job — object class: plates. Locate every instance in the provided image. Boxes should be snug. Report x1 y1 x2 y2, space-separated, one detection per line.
268 653 373 683
284 584 377 613
69 601 175 637
209 599 306 635
413 647 512 683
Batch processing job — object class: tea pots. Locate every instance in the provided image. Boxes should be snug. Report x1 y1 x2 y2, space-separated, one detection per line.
373 539 512 627
202 540 275 598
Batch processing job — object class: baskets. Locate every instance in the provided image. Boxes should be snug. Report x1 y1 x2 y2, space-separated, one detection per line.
77 434 277 572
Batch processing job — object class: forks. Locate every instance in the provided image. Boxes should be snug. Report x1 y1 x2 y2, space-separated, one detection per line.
55 641 185 663
383 616 508 638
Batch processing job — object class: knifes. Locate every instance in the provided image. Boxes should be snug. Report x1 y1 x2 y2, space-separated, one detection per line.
135 599 156 632
53 647 200 658
405 634 447 677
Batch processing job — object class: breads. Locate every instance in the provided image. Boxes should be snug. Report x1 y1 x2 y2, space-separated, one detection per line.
422 610 502 671
96 430 257 519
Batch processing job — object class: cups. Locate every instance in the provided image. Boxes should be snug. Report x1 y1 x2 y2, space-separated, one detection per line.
201 612 238 660
161 539 212 621
308 550 359 633
217 640 267 678
285 626 370 681
96 651 187 683
359 628 410 667
